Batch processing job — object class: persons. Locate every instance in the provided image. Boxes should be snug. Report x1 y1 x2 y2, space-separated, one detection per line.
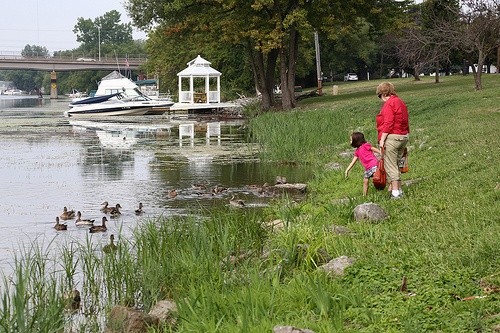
346 132 381 197
376 82 410 199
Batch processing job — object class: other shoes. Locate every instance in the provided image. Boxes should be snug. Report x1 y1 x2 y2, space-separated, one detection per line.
391 192 404 200
388 185 393 192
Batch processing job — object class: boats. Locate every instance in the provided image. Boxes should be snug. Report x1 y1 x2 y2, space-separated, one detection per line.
64 55 242 122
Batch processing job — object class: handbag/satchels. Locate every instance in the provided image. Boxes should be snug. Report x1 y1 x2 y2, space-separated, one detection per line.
397 147 409 173
373 147 387 191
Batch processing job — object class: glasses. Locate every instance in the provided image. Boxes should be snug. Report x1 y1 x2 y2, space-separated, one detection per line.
377 94 383 99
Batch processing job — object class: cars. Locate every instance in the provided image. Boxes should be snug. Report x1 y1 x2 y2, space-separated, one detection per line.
344 72 358 81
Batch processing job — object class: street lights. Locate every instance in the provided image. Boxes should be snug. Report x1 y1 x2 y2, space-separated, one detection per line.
97 25 102 62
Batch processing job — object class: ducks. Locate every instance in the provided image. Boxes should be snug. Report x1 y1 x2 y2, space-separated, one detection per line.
89 217 108 233
60 206 76 219
75 211 95 228
109 204 122 216
135 203 145 213
228 192 245 209
103 234 118 254
99 202 115 212
54 217 67 231
